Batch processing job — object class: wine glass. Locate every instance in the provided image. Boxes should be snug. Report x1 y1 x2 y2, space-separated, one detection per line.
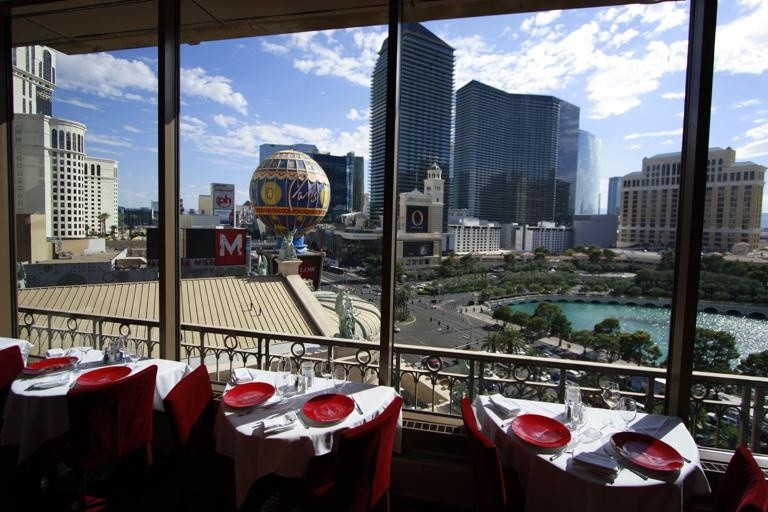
275 370 290 405
602 381 619 426
321 358 333 395
618 398 637 432
67 333 94 374
119 334 142 372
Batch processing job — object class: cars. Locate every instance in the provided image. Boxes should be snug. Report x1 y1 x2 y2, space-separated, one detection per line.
421 339 645 411
361 280 426 295
394 325 401 332
487 274 497 279
694 433 715 445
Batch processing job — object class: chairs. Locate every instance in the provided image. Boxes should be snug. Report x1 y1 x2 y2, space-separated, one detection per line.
163 362 275 510
56 366 161 510
281 395 404 511
457 396 514 509
1 345 26 400
686 446 768 510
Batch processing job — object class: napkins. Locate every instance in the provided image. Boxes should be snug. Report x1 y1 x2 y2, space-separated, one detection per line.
261 414 291 434
571 451 618 483
230 366 248 386
490 392 519 416
31 373 65 390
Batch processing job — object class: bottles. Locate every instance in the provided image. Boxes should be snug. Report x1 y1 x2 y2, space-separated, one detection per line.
294 361 313 395
103 339 124 364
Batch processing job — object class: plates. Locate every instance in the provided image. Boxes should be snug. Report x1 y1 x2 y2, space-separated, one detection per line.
610 430 684 472
224 382 275 408
303 393 356 424
512 413 571 448
23 356 78 377
75 367 132 388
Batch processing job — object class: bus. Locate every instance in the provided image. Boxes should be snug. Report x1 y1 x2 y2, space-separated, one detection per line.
327 266 345 275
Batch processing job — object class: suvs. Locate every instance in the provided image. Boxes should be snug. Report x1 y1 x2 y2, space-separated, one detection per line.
704 411 739 428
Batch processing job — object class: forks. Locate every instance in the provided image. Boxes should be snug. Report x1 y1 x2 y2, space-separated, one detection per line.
293 405 309 429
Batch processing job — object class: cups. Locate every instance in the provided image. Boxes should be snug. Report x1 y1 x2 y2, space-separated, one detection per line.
278 356 292 377
564 381 581 417
331 368 347 394
569 402 587 426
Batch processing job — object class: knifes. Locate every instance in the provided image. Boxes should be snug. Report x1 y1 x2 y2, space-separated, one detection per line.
611 456 648 479
550 438 579 461
